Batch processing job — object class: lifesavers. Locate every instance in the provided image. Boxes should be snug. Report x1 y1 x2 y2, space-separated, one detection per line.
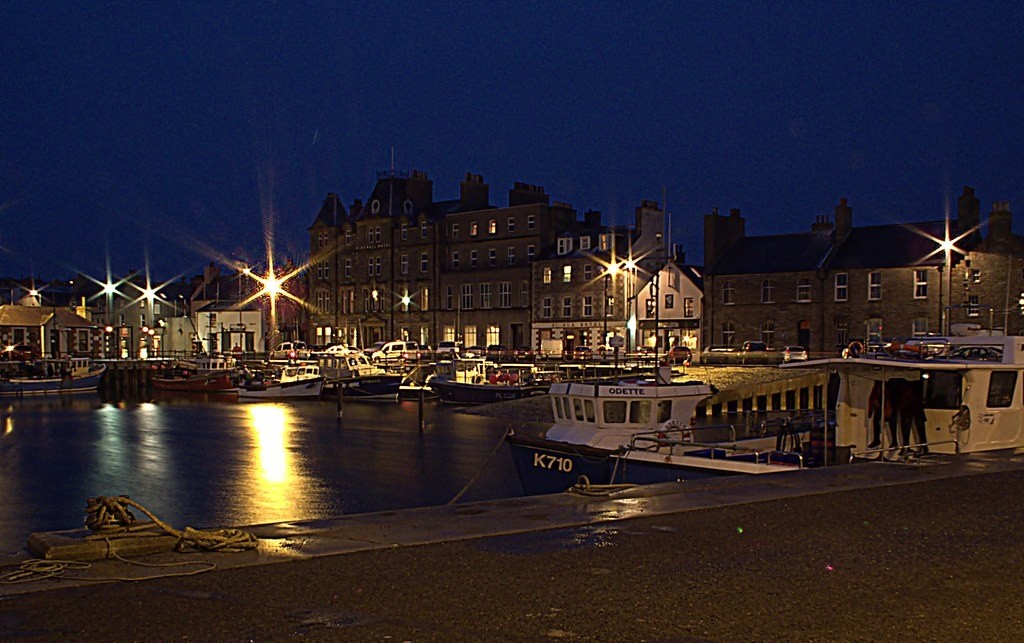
889 342 930 358
657 419 691 442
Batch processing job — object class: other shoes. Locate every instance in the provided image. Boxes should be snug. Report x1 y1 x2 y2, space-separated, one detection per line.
888 443 898 451
899 449 910 456
914 450 929 457
868 440 881 448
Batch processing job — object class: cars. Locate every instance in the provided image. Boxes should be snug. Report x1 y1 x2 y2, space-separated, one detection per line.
704 345 729 364
779 345 808 363
271 340 549 362
737 340 769 365
572 345 692 364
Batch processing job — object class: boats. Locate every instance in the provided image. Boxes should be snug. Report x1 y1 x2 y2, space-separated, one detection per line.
893 336 1024 364
776 355 1024 460
154 370 241 391
238 362 679 399
2 351 107 395
505 379 809 491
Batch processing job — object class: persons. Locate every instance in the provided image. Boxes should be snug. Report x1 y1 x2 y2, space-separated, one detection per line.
490 369 519 387
